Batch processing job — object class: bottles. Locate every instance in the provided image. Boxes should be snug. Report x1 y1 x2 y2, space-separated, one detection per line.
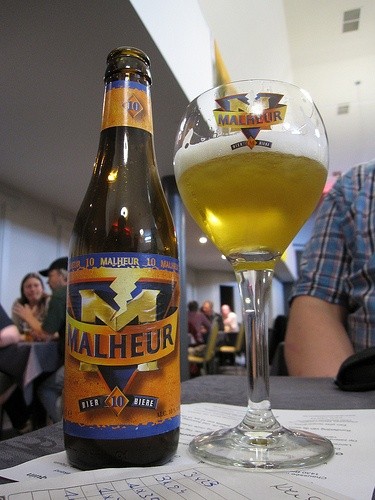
63 45 181 470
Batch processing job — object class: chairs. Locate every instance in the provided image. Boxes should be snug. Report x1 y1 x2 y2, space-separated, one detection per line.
219 324 245 365
188 320 218 363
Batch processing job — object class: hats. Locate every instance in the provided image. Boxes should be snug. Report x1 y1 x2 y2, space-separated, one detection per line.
39 256 69 276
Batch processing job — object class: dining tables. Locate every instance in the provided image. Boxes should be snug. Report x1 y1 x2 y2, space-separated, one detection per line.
216 331 239 346
0 374 375 500
0 341 62 405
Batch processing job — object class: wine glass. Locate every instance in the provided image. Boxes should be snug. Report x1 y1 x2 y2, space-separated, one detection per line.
172 78 335 473
